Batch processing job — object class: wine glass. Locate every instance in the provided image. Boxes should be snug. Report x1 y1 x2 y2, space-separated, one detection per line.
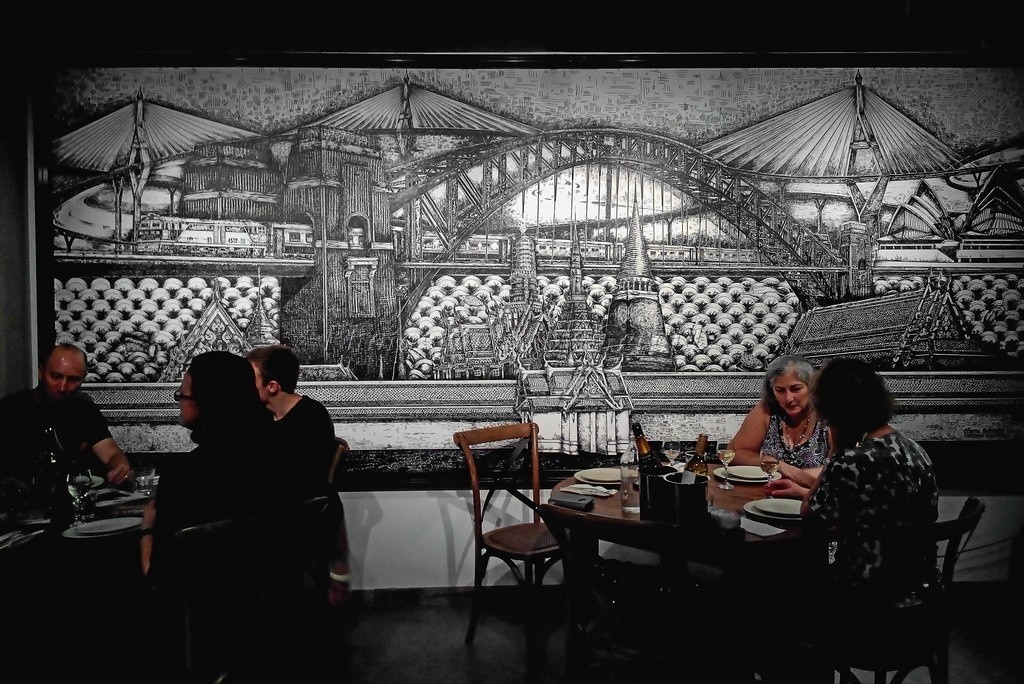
66 470 94 527
662 435 681 468
760 452 781 499
716 440 736 489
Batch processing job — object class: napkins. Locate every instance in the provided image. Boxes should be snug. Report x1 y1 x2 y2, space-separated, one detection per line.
559 481 617 497
734 514 786 538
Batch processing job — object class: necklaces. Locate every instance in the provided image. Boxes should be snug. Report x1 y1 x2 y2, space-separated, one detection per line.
786 417 810 447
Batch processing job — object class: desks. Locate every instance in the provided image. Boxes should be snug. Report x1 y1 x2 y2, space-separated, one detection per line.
549 461 852 684
0 469 190 684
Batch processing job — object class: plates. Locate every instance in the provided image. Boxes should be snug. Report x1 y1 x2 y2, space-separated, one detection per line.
574 468 636 485
713 465 782 483
742 498 813 521
74 476 104 488
73 516 145 534
63 524 141 538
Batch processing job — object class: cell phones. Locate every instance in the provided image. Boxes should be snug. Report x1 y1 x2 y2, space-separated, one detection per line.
549 492 594 512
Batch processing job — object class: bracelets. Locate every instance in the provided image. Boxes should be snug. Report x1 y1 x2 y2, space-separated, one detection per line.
141 528 154 538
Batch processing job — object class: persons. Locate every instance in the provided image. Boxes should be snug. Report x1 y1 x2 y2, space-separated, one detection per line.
141 345 336 594
0 344 132 485
728 355 833 467
762 359 939 609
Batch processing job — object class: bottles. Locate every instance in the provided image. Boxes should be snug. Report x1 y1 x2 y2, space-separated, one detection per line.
680 435 709 485
620 415 642 514
633 423 665 475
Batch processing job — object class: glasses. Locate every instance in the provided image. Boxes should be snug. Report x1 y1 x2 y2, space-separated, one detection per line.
174 390 194 403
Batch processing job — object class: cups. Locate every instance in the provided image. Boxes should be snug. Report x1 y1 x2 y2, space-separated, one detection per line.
685 452 707 466
664 472 710 528
138 466 155 495
636 465 677 521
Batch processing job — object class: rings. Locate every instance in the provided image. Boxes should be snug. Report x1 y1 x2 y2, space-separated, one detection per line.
125 476 128 478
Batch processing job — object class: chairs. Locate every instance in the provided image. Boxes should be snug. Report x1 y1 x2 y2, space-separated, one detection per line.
453 423 572 663
174 495 340 684
536 503 697 684
830 496 986 684
